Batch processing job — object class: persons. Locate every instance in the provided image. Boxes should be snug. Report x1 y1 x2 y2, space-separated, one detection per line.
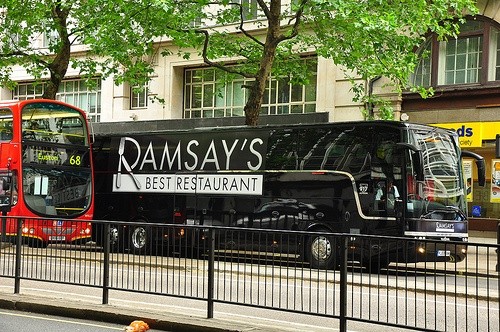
375 182 400 202
23 122 39 136
466 178 471 195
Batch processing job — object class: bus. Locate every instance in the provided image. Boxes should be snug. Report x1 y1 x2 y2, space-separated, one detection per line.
0 99 94 249
53 119 486 273
